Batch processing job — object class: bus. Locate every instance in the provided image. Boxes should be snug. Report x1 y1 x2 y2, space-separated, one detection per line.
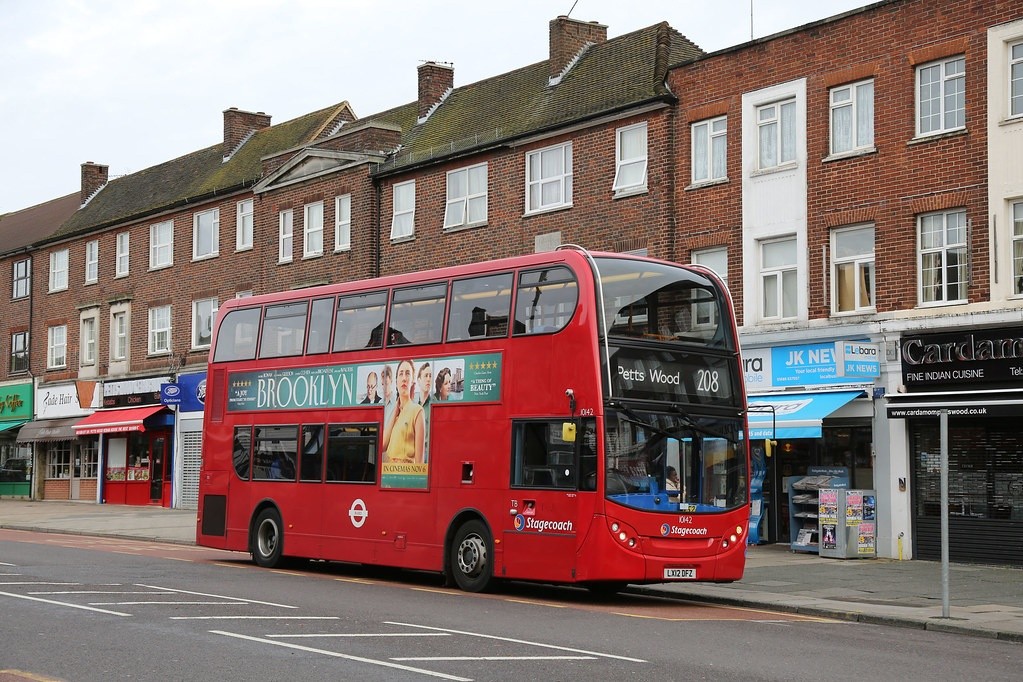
195 243 776 595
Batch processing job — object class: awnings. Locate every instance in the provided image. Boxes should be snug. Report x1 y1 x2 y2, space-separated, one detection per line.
636 390 863 441
0 421 25 433
17 418 97 443
882 385 1023 419
71 405 166 435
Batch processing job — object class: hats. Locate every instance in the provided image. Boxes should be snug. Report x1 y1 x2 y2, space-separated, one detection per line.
450 368 464 385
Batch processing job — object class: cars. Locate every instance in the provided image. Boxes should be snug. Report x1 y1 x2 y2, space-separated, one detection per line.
1 459 28 480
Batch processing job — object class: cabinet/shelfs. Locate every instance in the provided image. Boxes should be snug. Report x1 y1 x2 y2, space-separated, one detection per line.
787 466 849 553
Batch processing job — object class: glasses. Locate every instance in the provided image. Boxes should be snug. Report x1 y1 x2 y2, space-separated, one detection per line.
366 384 377 390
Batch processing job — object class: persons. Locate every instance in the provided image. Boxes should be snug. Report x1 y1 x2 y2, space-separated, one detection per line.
434 368 454 401
666 466 685 502
383 359 425 463
416 363 432 463
381 365 396 425
359 371 382 403
